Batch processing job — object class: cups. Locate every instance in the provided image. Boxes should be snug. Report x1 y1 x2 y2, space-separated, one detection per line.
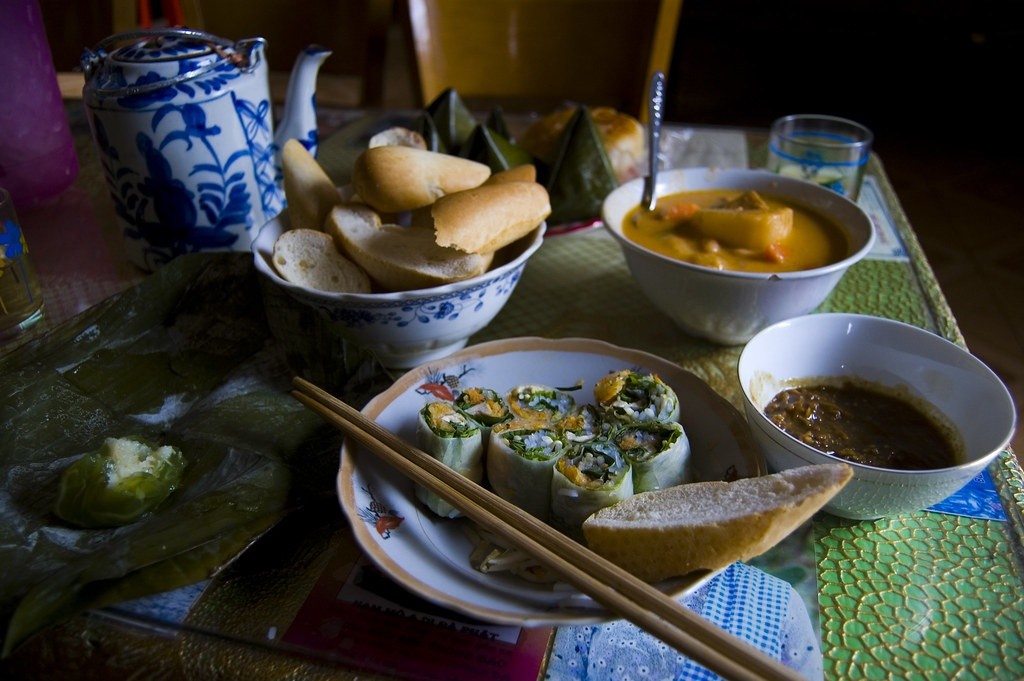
769 114 872 203
1 184 48 341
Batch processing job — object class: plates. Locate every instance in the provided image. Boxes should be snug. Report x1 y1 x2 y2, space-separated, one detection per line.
338 337 761 625
545 219 603 240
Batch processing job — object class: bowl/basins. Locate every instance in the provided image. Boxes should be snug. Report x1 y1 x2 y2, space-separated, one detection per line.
602 168 876 346
738 314 1016 521
252 186 547 366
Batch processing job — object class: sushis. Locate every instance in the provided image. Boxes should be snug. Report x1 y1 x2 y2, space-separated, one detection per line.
416 366 693 535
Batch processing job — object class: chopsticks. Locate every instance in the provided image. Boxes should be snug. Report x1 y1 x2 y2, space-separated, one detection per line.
290 374 810 681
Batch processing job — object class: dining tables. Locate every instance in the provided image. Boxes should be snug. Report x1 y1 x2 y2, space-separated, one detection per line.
0 119 1024 681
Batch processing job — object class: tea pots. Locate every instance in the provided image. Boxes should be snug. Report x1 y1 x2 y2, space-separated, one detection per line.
75 30 333 253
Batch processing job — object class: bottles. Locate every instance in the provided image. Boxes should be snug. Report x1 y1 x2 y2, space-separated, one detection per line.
1 0 136 325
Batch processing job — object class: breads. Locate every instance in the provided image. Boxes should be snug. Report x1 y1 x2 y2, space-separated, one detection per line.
271 125 551 293
519 104 649 180
582 465 856 581
